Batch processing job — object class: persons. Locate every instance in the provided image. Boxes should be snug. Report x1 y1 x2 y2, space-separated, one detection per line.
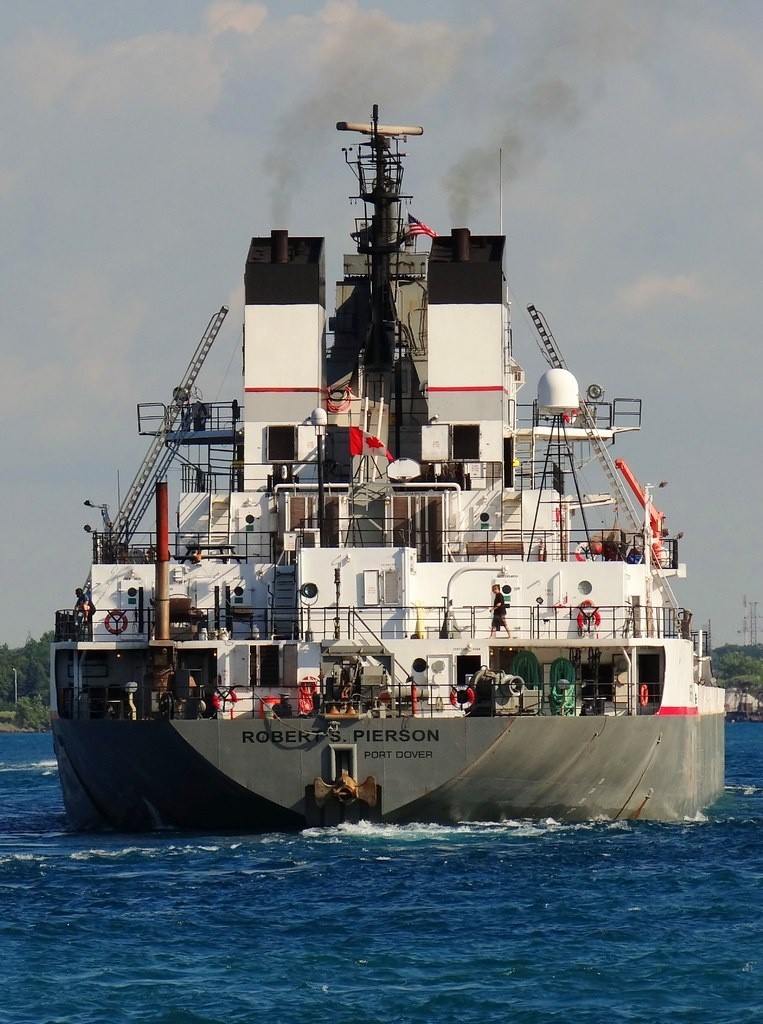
75 588 92 642
489 585 513 639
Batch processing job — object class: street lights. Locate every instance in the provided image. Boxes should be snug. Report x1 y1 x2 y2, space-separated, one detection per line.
12 668 18 708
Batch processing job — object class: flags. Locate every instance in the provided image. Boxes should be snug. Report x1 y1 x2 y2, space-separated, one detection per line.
350 426 394 461
408 214 437 238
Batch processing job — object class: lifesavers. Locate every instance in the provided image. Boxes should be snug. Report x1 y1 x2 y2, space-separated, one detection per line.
212 687 238 712
574 543 597 562
563 409 577 424
299 676 317 694
640 685 649 708
577 609 601 631
104 611 128 635
450 685 475 710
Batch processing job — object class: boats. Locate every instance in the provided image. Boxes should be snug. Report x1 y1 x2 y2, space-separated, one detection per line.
48 104 726 829
